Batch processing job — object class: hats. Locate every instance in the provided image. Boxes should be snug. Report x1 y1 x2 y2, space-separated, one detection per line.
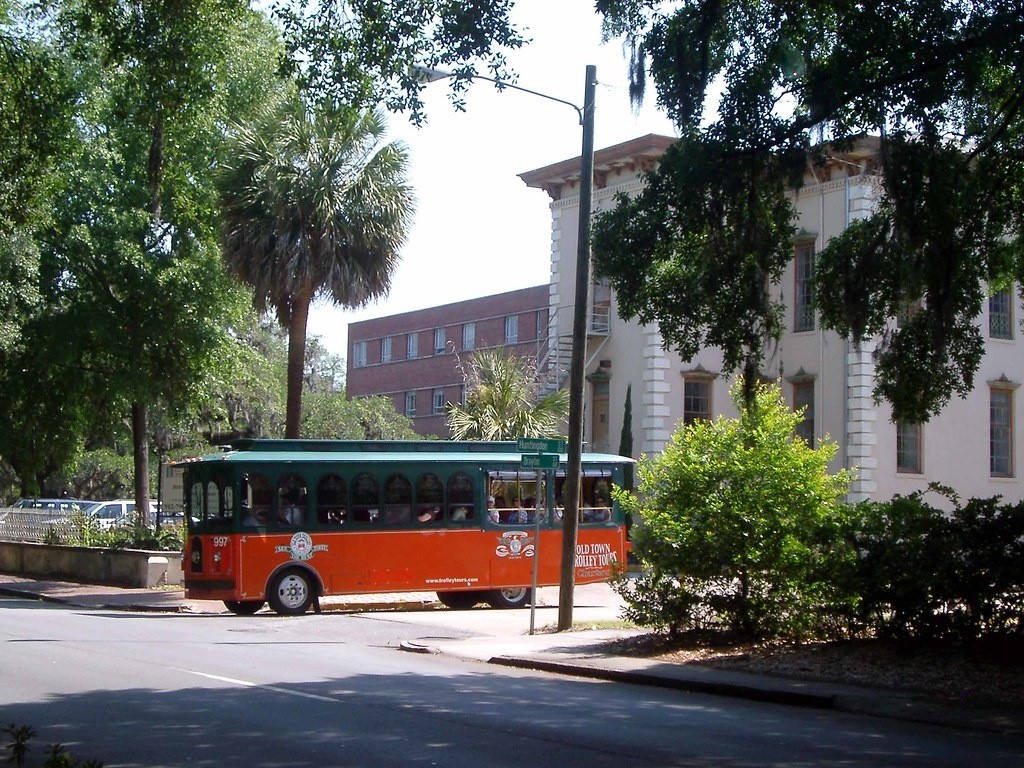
487 496 495 504
255 509 268 514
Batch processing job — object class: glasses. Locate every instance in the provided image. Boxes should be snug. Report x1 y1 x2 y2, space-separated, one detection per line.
597 500 603 503
514 500 518 502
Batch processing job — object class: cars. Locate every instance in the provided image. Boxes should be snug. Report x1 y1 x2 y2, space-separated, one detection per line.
9 498 201 538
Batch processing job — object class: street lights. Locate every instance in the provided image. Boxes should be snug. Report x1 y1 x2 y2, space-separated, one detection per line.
407 65 599 632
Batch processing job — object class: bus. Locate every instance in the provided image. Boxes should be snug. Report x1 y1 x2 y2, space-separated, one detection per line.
165 438 638 617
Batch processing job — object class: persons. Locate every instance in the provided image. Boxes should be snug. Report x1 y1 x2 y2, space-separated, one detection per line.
242 491 612 525
